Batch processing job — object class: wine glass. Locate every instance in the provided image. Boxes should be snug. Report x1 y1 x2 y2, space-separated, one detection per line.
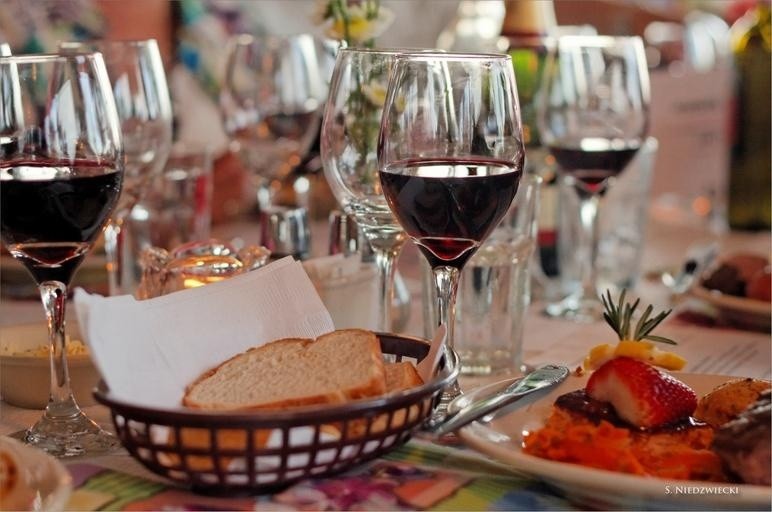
533 29 656 318
374 50 525 428
2 48 159 463
318 46 460 363
215 35 349 271
46 40 175 299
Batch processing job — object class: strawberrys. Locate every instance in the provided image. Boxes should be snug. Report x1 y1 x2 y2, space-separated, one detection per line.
584 355 698 432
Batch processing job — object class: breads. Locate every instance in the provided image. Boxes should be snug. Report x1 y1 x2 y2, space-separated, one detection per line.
522 377 771 486
1 446 45 512
166 328 432 469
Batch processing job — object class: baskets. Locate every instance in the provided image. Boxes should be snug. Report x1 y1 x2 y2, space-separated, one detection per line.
91 329 462 499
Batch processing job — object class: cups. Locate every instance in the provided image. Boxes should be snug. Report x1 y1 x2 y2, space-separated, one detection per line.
530 135 663 321
419 168 557 377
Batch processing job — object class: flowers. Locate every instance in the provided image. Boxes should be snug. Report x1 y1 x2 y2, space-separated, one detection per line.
313 1 407 193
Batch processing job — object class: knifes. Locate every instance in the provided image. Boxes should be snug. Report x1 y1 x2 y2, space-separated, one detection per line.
333 368 572 479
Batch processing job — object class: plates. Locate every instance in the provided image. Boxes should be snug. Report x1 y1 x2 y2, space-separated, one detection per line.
445 367 770 512
685 264 771 325
2 434 78 510
